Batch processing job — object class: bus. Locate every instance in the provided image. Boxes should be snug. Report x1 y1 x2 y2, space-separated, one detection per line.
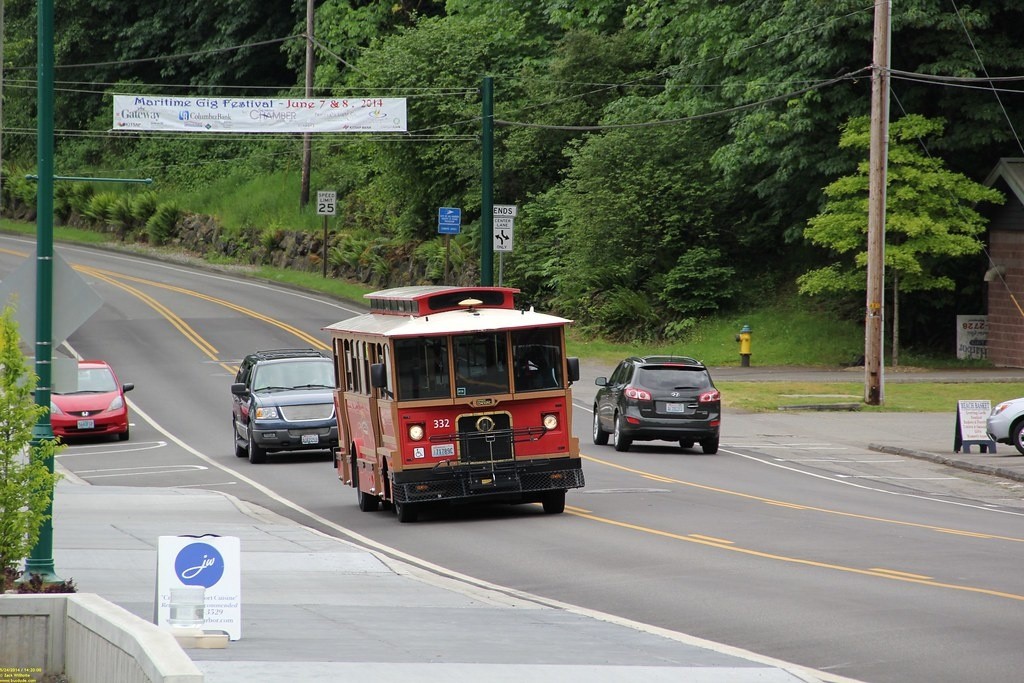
320 286 586 523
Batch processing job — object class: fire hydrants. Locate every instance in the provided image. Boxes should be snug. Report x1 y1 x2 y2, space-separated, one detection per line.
735 325 755 367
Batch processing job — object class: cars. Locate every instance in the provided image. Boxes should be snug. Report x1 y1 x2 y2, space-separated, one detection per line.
41 360 134 443
231 349 340 463
594 355 720 453
986 397 1023 455
